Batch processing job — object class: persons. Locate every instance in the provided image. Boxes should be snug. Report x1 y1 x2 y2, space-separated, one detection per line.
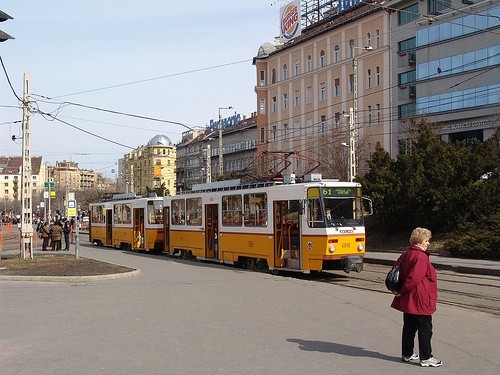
36 218 75 251
390 227 443 367
326 207 335 220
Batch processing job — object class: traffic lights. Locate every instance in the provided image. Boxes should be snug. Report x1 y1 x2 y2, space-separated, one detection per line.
0 9 16 42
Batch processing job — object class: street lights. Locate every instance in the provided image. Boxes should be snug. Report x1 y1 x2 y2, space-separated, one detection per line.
351 45 373 177
218 106 234 176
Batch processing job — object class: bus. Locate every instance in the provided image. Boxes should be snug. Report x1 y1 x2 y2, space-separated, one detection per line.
161 149 373 275
88 192 163 255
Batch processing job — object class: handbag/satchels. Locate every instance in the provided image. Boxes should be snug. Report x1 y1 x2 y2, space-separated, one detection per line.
385 251 406 291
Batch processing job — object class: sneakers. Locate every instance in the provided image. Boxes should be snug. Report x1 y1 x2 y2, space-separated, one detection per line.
402 352 419 362
420 357 442 367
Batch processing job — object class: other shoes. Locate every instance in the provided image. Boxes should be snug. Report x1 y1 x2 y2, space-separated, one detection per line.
63 248 69 251
